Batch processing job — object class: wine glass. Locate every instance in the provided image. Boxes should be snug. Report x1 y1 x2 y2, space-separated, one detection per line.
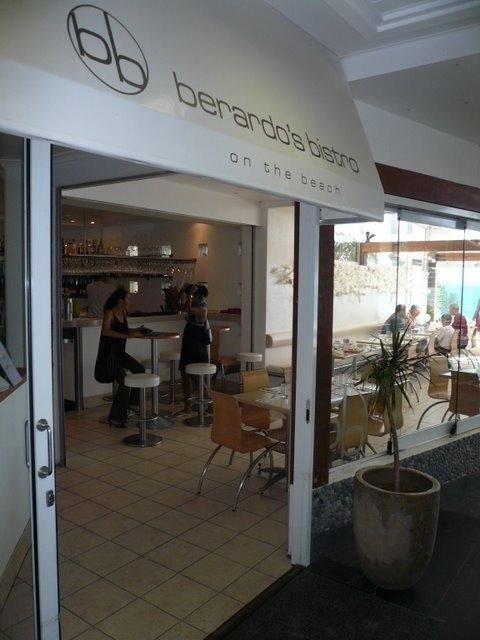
63 257 193 278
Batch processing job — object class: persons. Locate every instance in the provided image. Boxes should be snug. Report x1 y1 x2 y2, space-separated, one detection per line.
86 275 117 318
94 289 146 428
172 282 212 417
407 304 421 325
448 303 469 349
381 304 412 334
472 295 480 336
435 313 455 355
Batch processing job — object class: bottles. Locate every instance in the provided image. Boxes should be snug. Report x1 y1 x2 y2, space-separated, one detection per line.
63 239 86 254
88 238 103 255
67 298 73 321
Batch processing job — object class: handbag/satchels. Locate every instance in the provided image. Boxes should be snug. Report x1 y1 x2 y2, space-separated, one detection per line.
200 319 213 344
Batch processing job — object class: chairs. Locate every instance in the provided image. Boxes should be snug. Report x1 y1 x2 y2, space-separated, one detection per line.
446 330 462 355
334 395 376 459
228 369 288 479
425 337 440 357
193 388 264 508
367 382 407 452
403 338 422 369
415 349 451 430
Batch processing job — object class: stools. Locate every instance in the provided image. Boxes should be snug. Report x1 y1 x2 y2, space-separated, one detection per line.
187 361 215 428
93 376 124 428
119 370 161 449
239 349 264 373
156 346 184 411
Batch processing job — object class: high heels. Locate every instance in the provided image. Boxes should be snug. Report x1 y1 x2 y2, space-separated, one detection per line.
106 419 127 428
127 406 140 413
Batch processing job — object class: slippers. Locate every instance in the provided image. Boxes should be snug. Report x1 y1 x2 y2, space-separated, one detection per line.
174 411 192 417
198 411 215 417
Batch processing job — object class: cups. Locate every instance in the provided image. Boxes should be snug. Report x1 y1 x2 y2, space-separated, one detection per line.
162 245 171 257
281 370 293 398
73 299 80 318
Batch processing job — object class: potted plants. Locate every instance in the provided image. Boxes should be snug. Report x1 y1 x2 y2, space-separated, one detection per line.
334 321 448 590
